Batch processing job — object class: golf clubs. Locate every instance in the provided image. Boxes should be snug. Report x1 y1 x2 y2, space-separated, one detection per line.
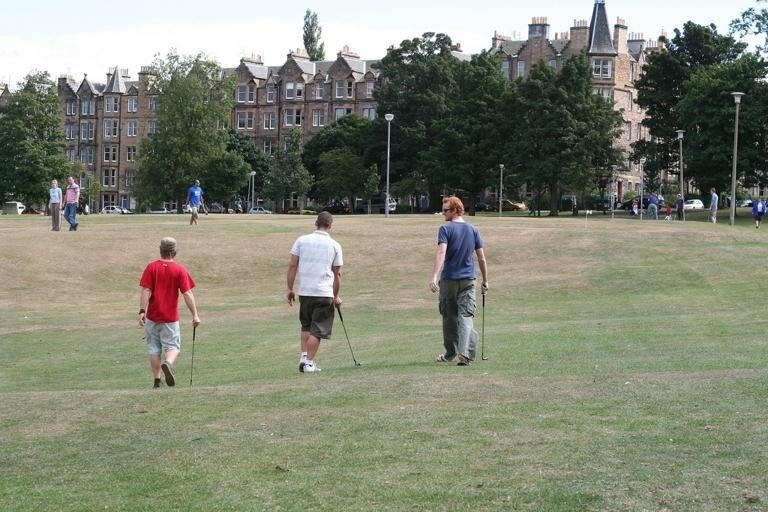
189 327 197 386
338 305 362 366
481 283 489 361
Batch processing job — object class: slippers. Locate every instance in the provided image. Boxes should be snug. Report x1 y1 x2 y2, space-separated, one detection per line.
457 355 469 365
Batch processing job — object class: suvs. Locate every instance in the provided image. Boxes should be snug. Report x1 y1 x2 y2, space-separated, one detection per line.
622 195 660 210
250 206 273 214
356 192 397 213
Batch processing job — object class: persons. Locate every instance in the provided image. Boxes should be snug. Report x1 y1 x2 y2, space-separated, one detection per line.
137 238 200 388
63 176 89 231
630 190 683 220
709 187 719 223
529 200 536 216
48 179 63 232
185 179 205 225
286 210 343 373
752 197 766 228
428 196 489 364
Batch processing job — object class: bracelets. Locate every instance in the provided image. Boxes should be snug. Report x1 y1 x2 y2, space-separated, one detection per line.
139 309 145 314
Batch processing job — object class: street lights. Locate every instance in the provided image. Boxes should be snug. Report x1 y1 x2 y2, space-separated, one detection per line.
250 170 256 207
246 173 250 213
639 158 646 221
610 164 616 217
675 129 685 222
383 113 394 218
728 92 745 226
498 163 504 213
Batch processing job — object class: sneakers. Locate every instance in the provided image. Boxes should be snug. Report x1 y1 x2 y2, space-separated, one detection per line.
161 363 175 386
437 354 456 362
299 356 321 373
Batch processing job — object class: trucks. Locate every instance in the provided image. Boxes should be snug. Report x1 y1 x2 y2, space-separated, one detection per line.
5 201 25 215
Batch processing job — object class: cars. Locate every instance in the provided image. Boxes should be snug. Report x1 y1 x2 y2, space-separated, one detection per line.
144 201 234 214
683 199 703 210
762 199 767 206
465 200 492 212
490 199 525 212
652 193 663 205
21 208 45 215
323 199 352 214
735 198 752 208
287 207 317 214
101 206 133 214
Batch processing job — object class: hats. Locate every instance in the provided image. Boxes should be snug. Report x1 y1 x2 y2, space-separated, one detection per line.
161 237 177 249
